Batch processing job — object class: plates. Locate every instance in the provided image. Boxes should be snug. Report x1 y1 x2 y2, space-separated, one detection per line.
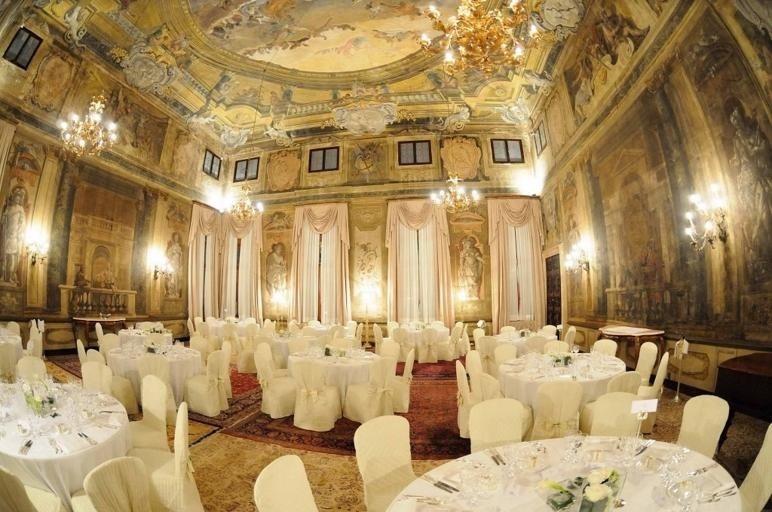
507 367 522 373
503 361 520 365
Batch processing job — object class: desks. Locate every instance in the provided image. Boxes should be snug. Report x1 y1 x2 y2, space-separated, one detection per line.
712 352 770 454
386 437 743 512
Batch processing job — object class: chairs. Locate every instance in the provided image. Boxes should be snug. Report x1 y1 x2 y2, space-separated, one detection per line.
1 310 234 512
228 309 670 442
468 399 527 457
352 414 418 512
676 394 730 460
737 423 771 512
253 454 319 512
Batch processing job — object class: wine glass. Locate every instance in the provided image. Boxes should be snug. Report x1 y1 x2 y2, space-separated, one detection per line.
451 427 701 512
522 342 605 381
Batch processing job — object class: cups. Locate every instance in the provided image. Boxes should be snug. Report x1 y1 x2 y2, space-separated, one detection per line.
152 344 169 360
0 375 109 437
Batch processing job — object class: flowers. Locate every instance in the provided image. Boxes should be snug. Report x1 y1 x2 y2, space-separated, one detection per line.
583 466 619 502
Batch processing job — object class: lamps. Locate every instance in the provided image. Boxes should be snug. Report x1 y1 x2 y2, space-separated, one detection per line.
415 0 547 80
683 183 731 252
52 88 120 165
428 57 482 216
226 69 266 221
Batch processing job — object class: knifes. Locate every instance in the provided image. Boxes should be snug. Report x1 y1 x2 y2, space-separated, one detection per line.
98 411 121 414
420 475 460 494
694 461 719 474
20 439 34 455
48 438 63 454
77 433 98 446
